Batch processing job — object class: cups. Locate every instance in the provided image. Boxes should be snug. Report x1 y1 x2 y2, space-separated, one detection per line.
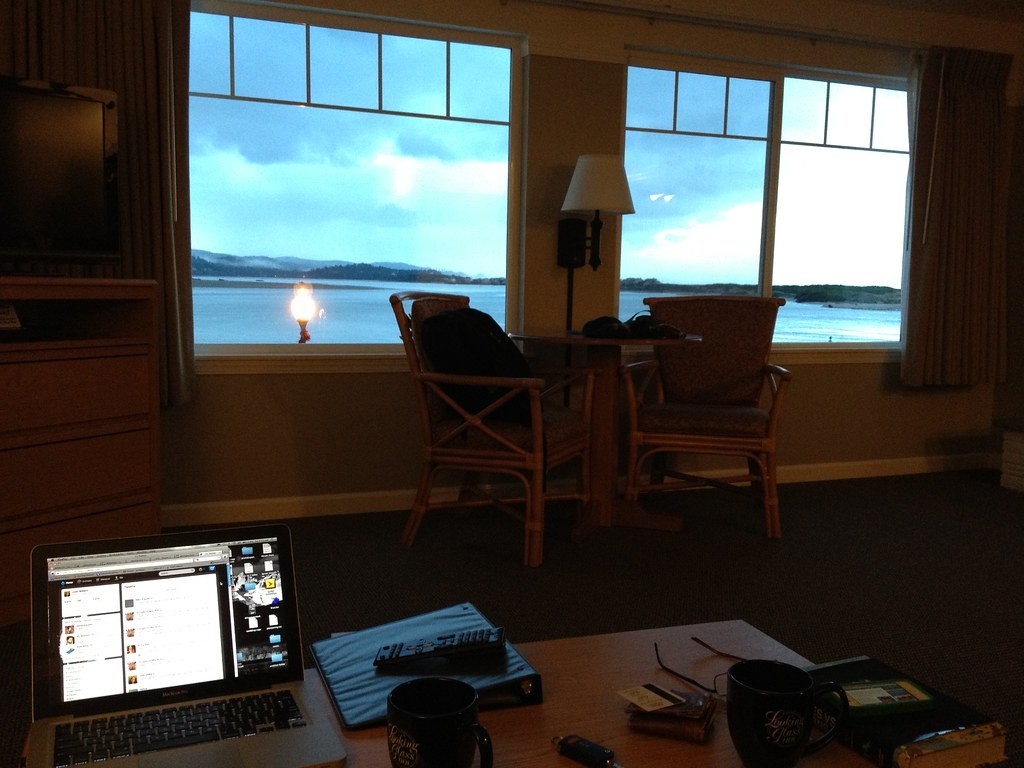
386 677 493 768
726 658 848 768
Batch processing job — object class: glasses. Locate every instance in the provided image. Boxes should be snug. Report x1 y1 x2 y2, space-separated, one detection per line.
654 637 749 696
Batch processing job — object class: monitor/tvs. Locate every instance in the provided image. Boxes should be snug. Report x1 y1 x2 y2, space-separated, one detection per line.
0 75 122 277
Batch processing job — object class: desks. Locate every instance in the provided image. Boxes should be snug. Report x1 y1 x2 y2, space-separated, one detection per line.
509 330 704 544
22 617 878 768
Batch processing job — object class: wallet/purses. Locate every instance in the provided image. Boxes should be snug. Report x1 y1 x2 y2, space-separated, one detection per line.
628 690 721 743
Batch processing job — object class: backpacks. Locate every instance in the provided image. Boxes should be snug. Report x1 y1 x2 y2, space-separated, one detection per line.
419 307 544 428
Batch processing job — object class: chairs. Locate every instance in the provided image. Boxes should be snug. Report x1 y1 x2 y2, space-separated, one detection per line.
391 292 597 568
620 296 792 540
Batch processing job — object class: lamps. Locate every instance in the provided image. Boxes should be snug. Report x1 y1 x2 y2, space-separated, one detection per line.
557 154 636 272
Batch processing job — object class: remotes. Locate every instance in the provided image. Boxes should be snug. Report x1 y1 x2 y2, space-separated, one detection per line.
373 627 507 667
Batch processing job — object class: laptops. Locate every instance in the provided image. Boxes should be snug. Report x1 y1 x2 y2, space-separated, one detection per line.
26 524 347 768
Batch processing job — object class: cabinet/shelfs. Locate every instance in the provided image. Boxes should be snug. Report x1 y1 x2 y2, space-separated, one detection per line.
0 277 163 629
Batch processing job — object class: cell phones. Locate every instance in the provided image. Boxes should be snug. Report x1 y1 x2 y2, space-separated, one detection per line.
823 677 936 718
